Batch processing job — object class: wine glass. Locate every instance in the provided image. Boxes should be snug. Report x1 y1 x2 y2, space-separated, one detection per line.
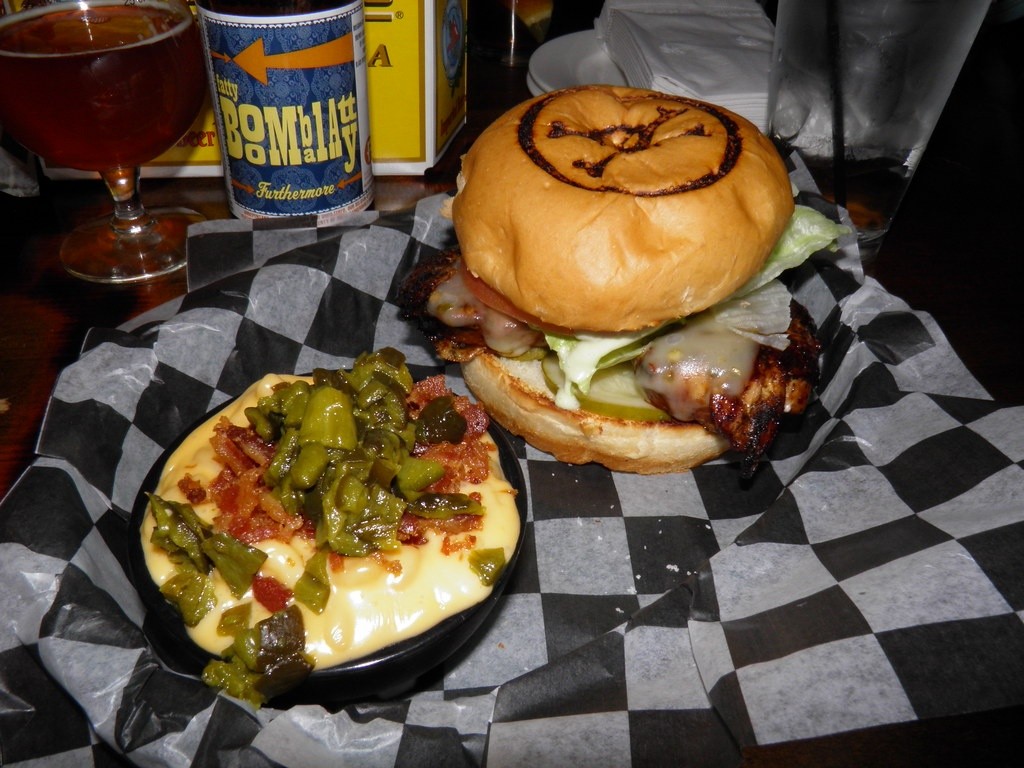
0 1 221 289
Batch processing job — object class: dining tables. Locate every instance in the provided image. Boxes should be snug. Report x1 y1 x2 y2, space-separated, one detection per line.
1 0 1024 768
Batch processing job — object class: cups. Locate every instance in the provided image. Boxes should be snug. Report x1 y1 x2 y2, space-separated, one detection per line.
757 1 987 247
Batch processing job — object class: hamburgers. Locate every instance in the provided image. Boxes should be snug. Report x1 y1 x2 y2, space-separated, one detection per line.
396 81 857 481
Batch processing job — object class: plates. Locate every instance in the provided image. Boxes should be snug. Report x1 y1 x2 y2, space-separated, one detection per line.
525 27 634 99
123 369 528 704
